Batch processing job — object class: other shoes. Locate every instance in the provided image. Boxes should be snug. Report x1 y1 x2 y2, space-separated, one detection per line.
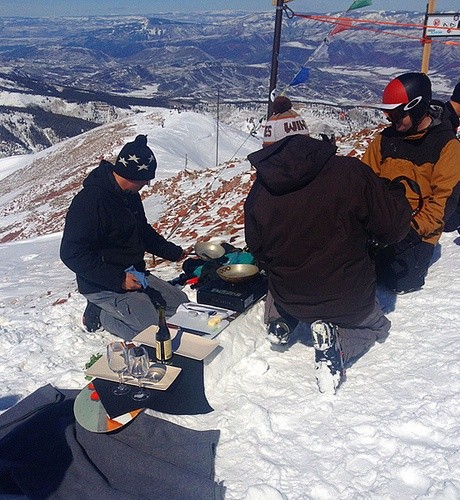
266 320 290 352
310 319 345 394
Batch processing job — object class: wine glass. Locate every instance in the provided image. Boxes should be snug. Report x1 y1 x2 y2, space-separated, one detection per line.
127 346 150 399
107 342 133 395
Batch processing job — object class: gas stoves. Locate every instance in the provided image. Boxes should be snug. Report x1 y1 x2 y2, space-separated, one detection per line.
196 277 268 313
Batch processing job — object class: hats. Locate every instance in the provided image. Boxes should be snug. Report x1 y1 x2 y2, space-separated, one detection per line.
450 82 460 104
114 134 157 182
262 96 310 148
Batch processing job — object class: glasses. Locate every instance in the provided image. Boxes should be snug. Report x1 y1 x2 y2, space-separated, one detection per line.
384 106 407 123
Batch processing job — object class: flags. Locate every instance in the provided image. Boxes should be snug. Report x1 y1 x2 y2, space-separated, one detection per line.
350 0 372 11
331 17 352 33
310 44 330 63
289 68 311 86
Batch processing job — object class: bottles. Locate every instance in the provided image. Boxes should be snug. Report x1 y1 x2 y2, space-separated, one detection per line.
154 306 174 366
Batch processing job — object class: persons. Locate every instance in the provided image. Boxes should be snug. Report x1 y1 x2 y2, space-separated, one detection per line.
59 134 189 343
357 74 460 292
439 81 460 236
243 96 414 396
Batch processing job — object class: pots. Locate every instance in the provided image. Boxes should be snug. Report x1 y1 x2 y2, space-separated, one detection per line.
202 252 259 284
185 241 226 259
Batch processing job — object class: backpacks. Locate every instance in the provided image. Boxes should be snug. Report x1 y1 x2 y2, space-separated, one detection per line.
367 174 424 263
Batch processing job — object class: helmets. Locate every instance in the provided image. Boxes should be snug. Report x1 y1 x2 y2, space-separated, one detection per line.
382 72 431 127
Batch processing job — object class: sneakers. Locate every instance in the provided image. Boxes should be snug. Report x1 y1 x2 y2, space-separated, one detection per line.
83 298 102 332
